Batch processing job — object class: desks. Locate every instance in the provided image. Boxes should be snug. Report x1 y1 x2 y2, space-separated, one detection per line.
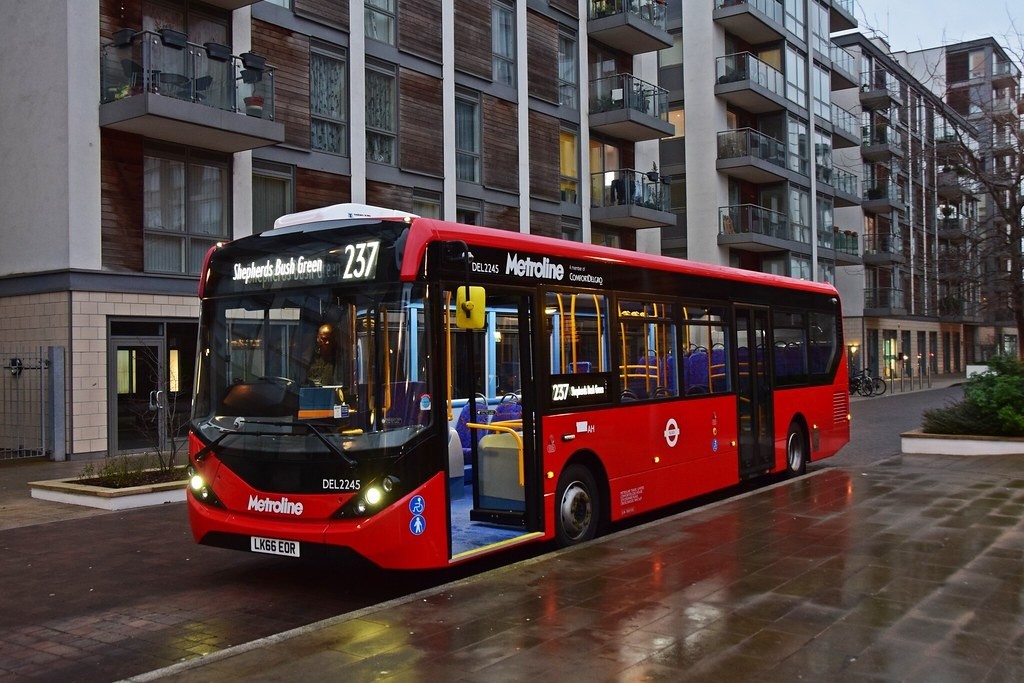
152 73 189 96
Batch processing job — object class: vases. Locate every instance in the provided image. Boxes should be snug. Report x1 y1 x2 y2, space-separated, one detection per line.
112 28 137 45
647 172 658 181
203 42 231 62
240 69 262 84
240 53 265 70
157 29 188 49
661 176 672 185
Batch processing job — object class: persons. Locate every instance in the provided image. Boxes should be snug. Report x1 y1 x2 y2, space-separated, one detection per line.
305 324 348 386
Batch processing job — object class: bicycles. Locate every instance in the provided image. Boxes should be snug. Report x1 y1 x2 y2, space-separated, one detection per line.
849 368 887 397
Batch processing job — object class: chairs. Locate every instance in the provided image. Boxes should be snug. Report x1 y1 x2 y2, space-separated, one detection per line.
456 339 832 447
121 58 161 87
177 75 213 102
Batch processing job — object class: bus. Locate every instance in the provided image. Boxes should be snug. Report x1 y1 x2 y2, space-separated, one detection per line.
186 202 850 585
